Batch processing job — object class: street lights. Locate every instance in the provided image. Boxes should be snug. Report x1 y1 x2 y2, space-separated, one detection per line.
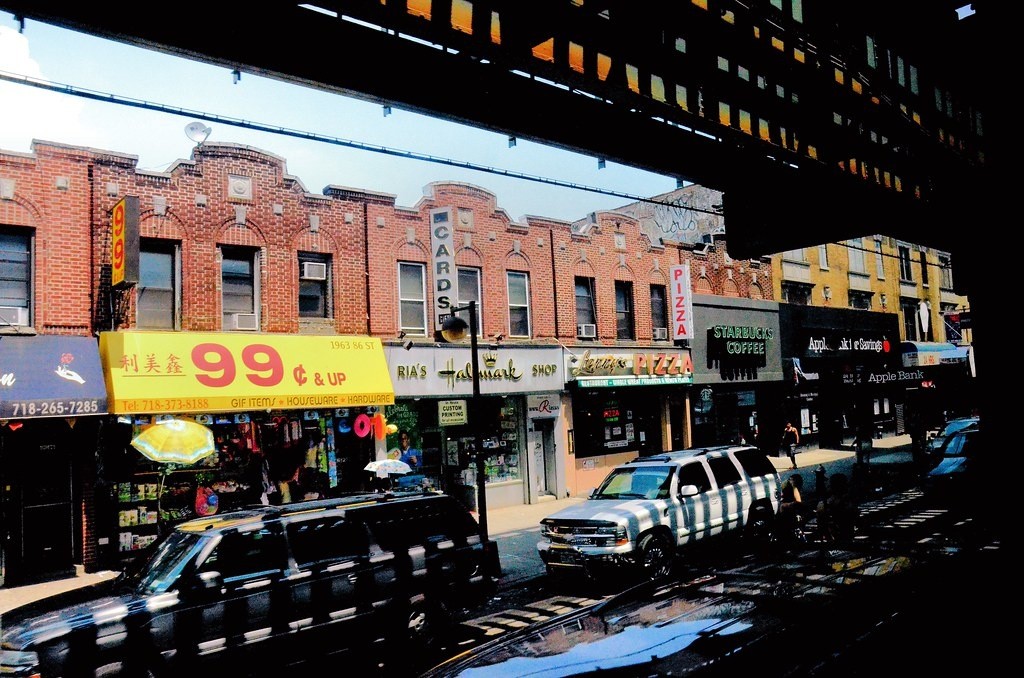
442 299 501 590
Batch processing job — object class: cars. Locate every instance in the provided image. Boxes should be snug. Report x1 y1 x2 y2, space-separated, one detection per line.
920 417 984 491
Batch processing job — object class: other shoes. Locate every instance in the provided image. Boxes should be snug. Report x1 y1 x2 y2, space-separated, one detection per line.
793 465 796 469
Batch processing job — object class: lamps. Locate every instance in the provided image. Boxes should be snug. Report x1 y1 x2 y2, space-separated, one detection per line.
554 338 578 363
402 339 413 351
494 332 503 342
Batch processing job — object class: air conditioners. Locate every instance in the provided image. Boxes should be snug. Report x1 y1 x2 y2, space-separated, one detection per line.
0 306 22 325
231 314 258 331
653 328 667 339
300 262 326 280
577 324 596 337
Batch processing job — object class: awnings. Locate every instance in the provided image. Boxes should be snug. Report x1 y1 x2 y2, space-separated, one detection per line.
900 339 959 368
97 328 393 417
566 371 694 390
0 334 109 421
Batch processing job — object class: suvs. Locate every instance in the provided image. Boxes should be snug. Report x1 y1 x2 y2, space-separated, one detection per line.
1 489 494 678
537 443 785 586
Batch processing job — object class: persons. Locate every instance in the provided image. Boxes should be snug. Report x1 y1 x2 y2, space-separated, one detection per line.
774 474 804 524
410 473 435 492
877 421 883 439
781 422 799 470
375 477 393 494
850 425 872 464
399 432 423 470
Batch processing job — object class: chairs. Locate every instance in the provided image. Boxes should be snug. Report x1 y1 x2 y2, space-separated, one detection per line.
257 533 286 572
216 532 247 577
631 475 657 494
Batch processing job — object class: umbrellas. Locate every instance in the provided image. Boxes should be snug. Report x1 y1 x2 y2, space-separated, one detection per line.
364 458 413 479
130 416 216 511
